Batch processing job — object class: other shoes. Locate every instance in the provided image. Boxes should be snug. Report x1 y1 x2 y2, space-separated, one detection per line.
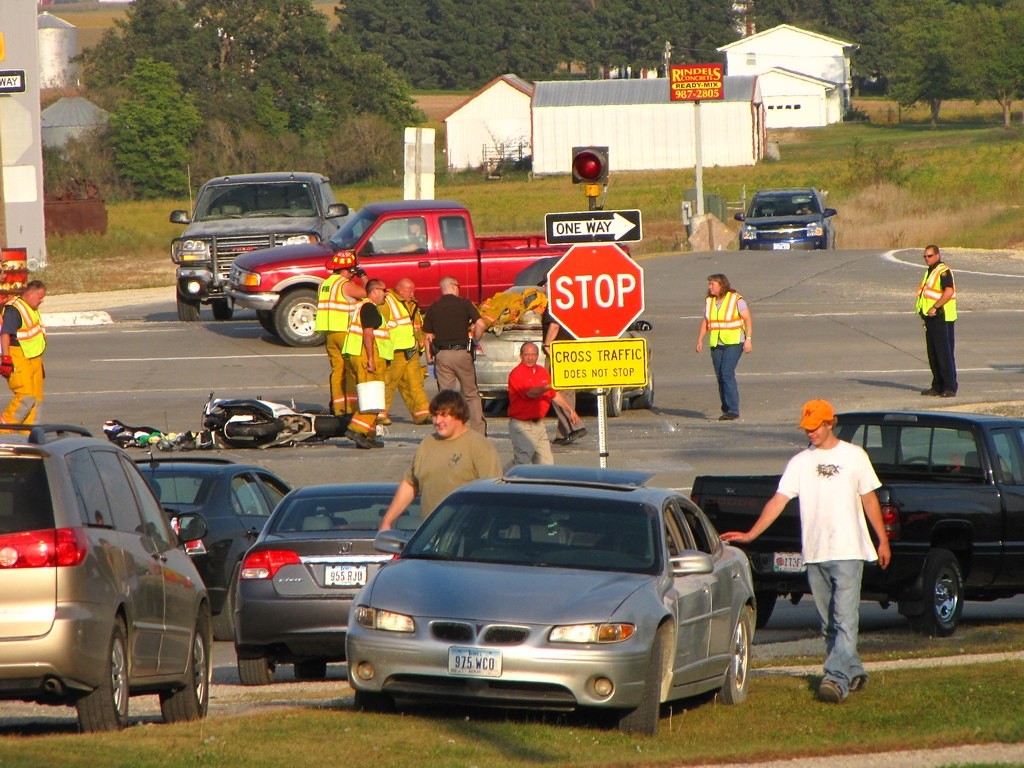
719 415 725 420
723 413 739 420
850 676 867 691
941 390 956 397
554 438 566 444
819 680 846 704
344 429 372 449
921 386 944 395
357 440 384 448
561 428 587 445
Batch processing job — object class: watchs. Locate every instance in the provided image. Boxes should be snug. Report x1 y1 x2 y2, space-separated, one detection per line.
569 409 574 414
934 306 939 309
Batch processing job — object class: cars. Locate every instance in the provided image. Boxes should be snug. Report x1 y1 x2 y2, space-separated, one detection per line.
345 465 756 738
233 483 423 687
458 286 656 417
132 456 348 642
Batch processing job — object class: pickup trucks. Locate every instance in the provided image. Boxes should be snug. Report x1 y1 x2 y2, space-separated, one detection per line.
169 172 363 321
224 199 630 347
691 411 1023 638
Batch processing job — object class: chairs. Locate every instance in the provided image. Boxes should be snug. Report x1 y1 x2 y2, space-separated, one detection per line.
761 204 777 216
302 516 333 531
396 515 421 530
866 446 896 464
221 202 246 214
488 514 532 551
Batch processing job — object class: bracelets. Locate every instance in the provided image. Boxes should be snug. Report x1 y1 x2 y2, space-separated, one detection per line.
746 336 752 340
359 271 366 278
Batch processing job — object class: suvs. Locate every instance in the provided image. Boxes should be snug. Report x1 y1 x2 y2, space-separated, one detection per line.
735 187 837 250
0 423 213 735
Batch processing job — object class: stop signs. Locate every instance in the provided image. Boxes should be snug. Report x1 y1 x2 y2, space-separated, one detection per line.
547 244 644 340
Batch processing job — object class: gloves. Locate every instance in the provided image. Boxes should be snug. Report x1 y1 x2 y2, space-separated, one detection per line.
0 355 14 379
42 365 45 378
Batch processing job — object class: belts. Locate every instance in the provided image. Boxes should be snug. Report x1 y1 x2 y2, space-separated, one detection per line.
439 345 466 350
532 419 538 422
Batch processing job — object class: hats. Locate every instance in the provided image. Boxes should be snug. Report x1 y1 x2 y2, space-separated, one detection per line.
798 399 833 430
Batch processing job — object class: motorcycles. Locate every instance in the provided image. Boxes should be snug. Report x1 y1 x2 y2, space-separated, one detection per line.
200 391 350 449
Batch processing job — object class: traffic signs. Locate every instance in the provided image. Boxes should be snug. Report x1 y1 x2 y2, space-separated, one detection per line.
0 69 26 95
544 209 642 245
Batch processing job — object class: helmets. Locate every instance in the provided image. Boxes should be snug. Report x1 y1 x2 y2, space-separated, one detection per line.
333 250 356 272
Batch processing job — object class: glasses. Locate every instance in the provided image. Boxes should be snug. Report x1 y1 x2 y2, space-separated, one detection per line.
924 255 932 257
376 287 386 293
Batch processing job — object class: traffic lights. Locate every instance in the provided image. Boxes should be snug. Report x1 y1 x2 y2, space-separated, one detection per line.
571 146 609 185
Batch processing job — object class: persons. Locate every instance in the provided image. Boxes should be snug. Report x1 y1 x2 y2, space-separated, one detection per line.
0 280 48 435
380 218 427 254
916 245 958 398
696 274 752 420
376 278 432 425
341 280 394 448
537 270 587 445
421 277 487 437
314 252 368 416
720 400 891 703
508 341 579 465
378 390 503 531
95 510 137 677
796 203 816 215
